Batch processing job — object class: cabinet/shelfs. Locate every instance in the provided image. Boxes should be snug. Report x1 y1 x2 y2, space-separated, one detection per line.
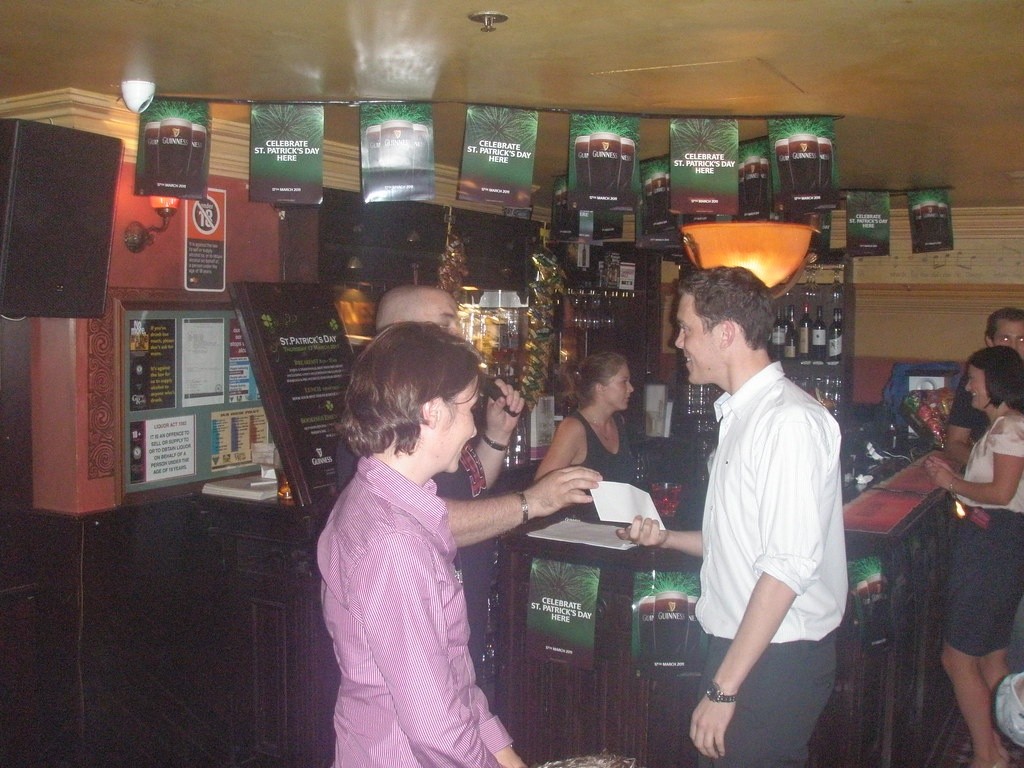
542 242 665 426
293 195 546 491
672 248 856 485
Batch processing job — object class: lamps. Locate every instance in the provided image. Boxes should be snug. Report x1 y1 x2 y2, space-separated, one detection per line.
678 211 821 297
124 196 178 253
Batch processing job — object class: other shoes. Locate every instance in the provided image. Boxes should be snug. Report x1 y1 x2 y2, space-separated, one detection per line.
994 747 1010 768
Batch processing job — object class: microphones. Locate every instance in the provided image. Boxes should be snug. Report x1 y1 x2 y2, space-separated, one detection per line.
480 377 519 417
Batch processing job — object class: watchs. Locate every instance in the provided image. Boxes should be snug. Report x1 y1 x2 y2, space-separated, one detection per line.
706 681 736 703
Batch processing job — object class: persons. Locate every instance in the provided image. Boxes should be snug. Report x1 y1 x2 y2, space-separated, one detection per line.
534 352 633 527
946 308 1024 465
317 321 528 768
617 266 848 768
925 345 1024 768
335 284 603 684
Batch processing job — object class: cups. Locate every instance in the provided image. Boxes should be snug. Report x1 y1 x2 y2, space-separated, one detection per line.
914 200 949 246
146 118 207 197
775 135 831 203
1011 672 1024 731
645 173 673 226
737 156 770 212
554 187 568 223
638 591 701 674
650 481 683 517
575 132 635 208
857 574 887 644
366 120 429 200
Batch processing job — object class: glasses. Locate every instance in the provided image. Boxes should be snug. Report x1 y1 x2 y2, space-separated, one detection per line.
992 336 1024 344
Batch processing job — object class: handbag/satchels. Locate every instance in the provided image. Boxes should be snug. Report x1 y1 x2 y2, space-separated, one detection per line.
621 415 648 487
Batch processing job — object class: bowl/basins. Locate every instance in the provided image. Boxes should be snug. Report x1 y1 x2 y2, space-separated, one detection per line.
680 222 823 301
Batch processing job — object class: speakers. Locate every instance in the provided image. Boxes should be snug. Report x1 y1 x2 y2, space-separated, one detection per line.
0 118 126 318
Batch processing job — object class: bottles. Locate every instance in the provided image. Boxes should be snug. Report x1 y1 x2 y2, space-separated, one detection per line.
682 384 722 416
789 375 842 417
479 593 499 704
563 289 640 330
694 419 719 482
767 304 842 367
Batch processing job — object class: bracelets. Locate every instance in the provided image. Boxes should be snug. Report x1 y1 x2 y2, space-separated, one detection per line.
516 492 529 524
950 479 954 489
482 433 508 451
